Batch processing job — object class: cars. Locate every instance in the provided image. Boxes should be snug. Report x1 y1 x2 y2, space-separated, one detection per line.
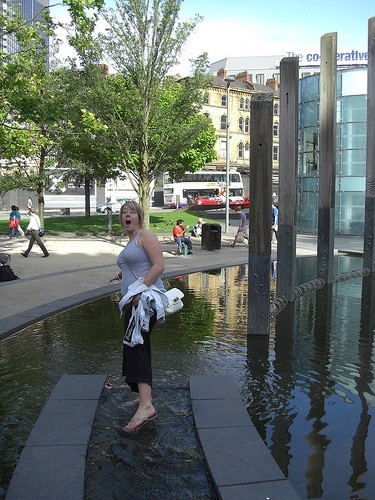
229 194 251 212
194 194 218 206
96 198 133 214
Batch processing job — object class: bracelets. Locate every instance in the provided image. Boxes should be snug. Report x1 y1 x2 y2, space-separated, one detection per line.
117 273 120 279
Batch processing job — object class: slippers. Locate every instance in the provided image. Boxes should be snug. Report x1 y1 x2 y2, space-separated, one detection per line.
125 414 157 432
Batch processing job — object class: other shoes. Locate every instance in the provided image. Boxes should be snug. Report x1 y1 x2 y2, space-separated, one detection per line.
230 245 234 247
21 252 28 257
42 253 50 258
188 251 193 255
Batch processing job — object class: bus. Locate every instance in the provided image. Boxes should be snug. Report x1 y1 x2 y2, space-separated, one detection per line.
161 170 245 209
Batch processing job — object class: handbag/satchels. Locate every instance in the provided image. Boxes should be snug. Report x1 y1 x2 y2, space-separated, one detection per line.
39 229 44 237
0 264 17 282
235 231 244 244
164 287 184 314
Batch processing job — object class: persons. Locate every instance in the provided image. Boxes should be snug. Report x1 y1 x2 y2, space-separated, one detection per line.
26 197 32 216
7 205 24 239
21 208 49 258
108 200 166 430
272 202 278 243
228 207 249 247
192 218 206 237
173 220 193 256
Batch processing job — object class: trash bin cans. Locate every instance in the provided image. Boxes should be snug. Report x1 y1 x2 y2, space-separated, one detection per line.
200 223 222 250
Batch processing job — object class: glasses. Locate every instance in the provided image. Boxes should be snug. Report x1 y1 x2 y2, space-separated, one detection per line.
120 210 138 215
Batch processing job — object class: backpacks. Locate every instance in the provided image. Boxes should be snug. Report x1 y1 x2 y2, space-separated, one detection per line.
0 253 12 265
